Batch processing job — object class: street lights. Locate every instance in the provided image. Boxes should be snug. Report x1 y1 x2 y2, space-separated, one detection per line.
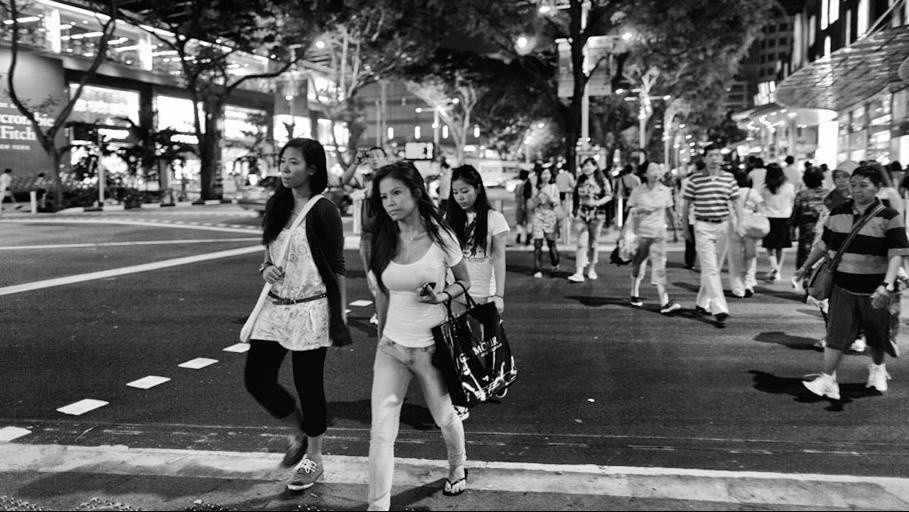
514 0 632 175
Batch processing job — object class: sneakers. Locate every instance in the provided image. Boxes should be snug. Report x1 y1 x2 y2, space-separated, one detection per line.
286 459 324 490
568 273 586 282
660 301 681 314
630 296 643 307
866 366 888 392
588 268 598 279
695 268 781 323
533 263 560 279
282 432 305 467
802 374 841 399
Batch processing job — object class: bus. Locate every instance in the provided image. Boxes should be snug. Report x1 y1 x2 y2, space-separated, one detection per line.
472 158 535 189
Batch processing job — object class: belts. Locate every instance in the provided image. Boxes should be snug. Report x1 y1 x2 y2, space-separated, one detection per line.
695 216 724 224
268 290 327 305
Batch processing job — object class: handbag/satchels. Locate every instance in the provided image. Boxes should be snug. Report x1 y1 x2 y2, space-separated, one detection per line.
808 254 837 300
239 282 272 344
609 239 632 267
743 212 771 238
430 301 518 408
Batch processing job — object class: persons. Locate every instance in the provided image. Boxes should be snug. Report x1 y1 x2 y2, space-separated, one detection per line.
359 162 472 512
32 172 48 209
244 139 352 492
0 167 23 210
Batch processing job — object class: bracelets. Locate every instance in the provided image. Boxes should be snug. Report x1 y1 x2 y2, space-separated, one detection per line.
258 262 271 274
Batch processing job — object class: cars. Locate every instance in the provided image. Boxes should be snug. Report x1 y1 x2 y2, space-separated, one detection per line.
236 172 354 221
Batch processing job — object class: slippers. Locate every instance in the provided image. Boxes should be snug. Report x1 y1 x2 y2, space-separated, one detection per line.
443 467 468 495
